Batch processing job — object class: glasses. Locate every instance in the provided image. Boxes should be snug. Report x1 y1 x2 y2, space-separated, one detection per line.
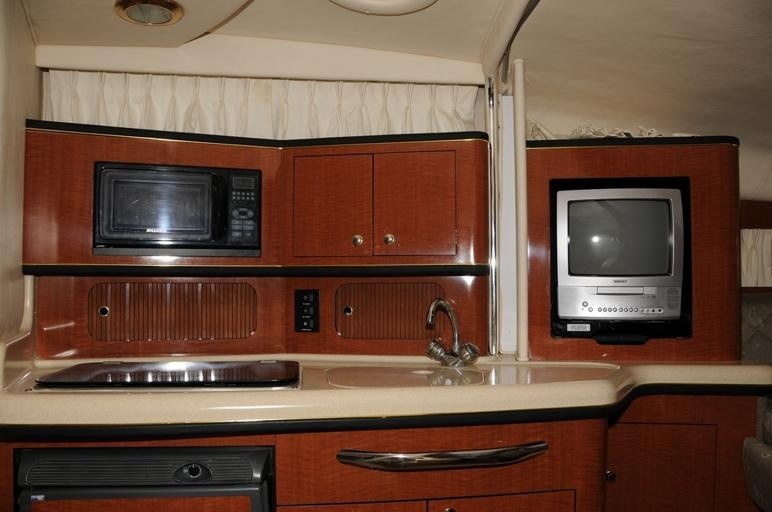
114 0 184 27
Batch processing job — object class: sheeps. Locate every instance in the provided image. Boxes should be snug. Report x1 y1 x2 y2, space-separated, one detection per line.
426 298 479 367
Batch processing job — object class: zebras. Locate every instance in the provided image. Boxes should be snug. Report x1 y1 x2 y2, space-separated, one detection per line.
12 445 274 510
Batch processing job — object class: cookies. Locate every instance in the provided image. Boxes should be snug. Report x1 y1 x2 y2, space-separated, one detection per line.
91 162 262 258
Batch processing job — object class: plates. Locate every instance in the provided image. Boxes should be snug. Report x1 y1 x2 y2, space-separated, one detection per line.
606 395 760 511
273 424 604 511
285 138 487 264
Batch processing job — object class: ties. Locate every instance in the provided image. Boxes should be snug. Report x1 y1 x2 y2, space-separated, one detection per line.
556 188 685 320
